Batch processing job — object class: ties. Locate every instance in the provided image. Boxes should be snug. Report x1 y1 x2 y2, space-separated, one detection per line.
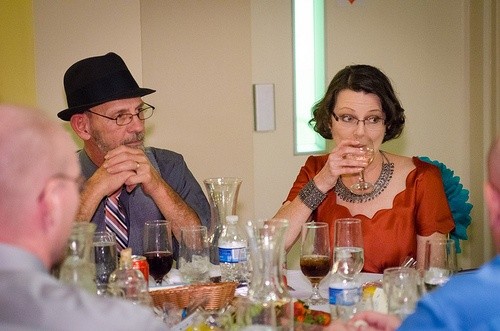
104 184 129 260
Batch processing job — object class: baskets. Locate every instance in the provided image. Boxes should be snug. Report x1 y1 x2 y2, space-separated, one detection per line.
144 282 236 312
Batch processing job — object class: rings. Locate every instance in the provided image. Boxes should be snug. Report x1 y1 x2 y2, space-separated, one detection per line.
135 161 140 170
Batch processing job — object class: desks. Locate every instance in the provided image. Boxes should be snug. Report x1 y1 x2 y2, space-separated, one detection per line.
286 269 383 311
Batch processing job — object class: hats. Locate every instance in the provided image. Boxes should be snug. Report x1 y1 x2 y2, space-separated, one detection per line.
57 53 156 120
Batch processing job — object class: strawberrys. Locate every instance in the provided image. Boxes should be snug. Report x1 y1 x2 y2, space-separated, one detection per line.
277 299 332 331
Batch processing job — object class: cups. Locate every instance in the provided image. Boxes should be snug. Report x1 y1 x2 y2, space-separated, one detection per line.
383 267 419 315
91 232 118 297
423 238 455 292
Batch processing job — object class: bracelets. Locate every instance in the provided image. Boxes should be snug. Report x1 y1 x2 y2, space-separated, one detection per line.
298 178 329 211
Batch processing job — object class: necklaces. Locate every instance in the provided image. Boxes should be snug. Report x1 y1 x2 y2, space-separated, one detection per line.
331 149 394 203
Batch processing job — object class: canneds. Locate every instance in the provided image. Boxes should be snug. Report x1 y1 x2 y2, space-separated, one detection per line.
130 255 148 298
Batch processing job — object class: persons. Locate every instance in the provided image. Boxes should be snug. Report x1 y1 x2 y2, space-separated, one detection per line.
47 52 213 292
319 136 500 331
0 102 170 330
262 64 457 278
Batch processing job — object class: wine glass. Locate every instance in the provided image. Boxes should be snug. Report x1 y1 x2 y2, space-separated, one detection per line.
345 135 375 196
332 217 365 282
178 226 210 284
299 222 332 304
142 220 174 286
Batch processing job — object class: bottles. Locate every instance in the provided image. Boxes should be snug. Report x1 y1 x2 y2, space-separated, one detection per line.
328 250 363 321
57 222 97 297
237 219 294 331
203 177 243 286
217 215 250 297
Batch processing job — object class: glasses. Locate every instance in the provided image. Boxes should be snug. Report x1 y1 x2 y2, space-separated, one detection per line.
86 102 155 125
38 173 87 201
331 110 386 126
